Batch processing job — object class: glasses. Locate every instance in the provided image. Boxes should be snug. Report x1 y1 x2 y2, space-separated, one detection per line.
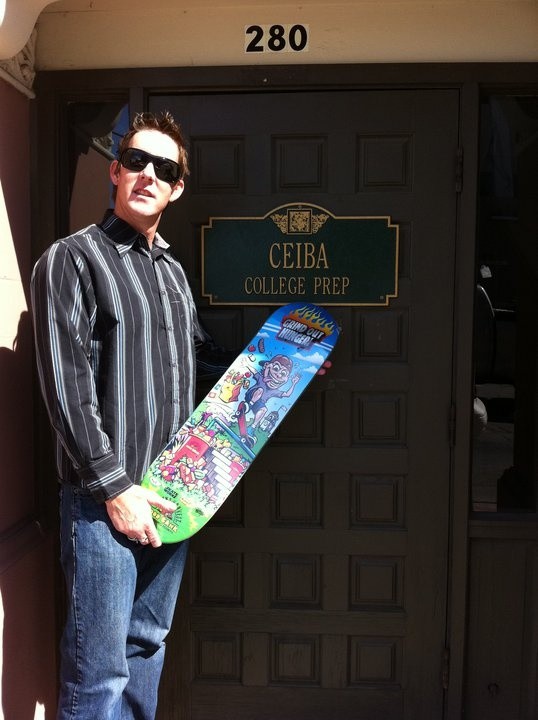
115 148 181 188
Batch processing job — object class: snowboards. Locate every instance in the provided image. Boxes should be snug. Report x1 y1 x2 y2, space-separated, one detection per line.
138 306 340 547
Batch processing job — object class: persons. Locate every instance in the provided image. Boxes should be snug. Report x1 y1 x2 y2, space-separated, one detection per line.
27 109 210 720
